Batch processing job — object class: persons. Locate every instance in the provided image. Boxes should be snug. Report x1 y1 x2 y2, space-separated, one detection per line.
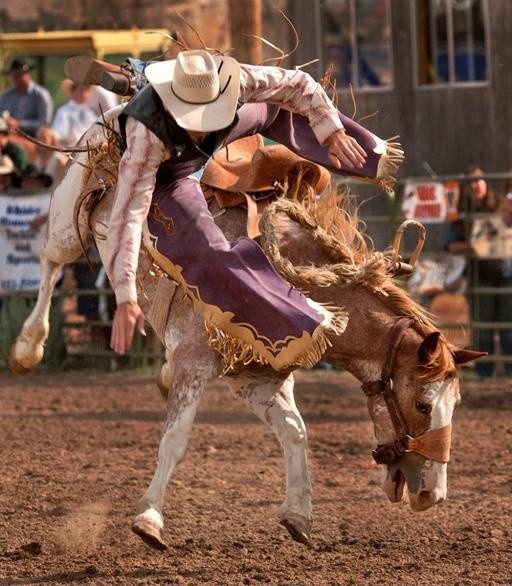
442 160 511 378
65 47 404 374
23 119 66 191
60 43 118 116
0 118 31 192
1 56 52 159
53 81 100 149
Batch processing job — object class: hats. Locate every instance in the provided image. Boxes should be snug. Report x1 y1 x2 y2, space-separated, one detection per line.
1 56 41 76
145 50 240 132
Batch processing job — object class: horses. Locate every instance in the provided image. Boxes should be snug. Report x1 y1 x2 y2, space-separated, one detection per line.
7 101 489 552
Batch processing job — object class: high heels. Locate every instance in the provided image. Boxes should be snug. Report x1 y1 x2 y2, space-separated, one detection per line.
64 54 137 95
